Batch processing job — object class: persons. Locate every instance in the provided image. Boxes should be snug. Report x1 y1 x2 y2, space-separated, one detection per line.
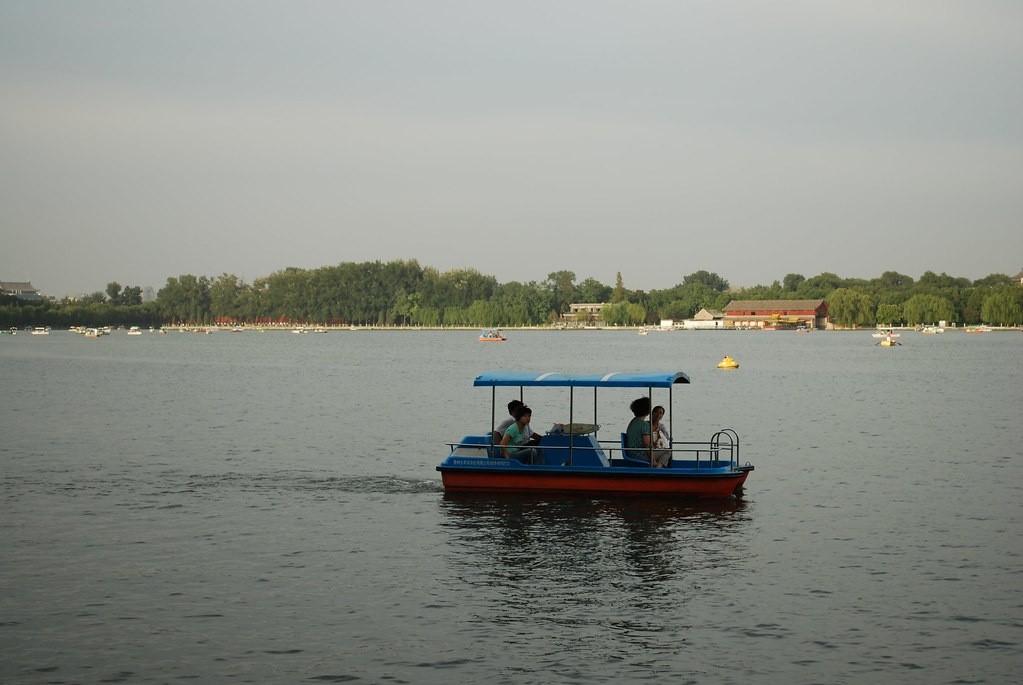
500 406 537 464
626 397 672 468
494 400 542 444
644 406 670 446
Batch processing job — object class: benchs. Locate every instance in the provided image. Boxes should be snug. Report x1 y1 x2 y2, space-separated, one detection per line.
621 433 650 465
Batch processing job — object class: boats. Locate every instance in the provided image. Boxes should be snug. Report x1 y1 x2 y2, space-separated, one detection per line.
479 333 507 342
149 325 155 333
68 325 112 338
292 327 309 334
231 325 243 332
638 328 649 336
717 355 740 369
796 326 813 333
879 335 897 347
31 327 49 335
914 323 945 335
314 329 328 333
436 369 755 504
178 325 213 335
127 325 143 336
10 327 17 335
966 325 992 335
158 328 167 335
871 327 902 339
